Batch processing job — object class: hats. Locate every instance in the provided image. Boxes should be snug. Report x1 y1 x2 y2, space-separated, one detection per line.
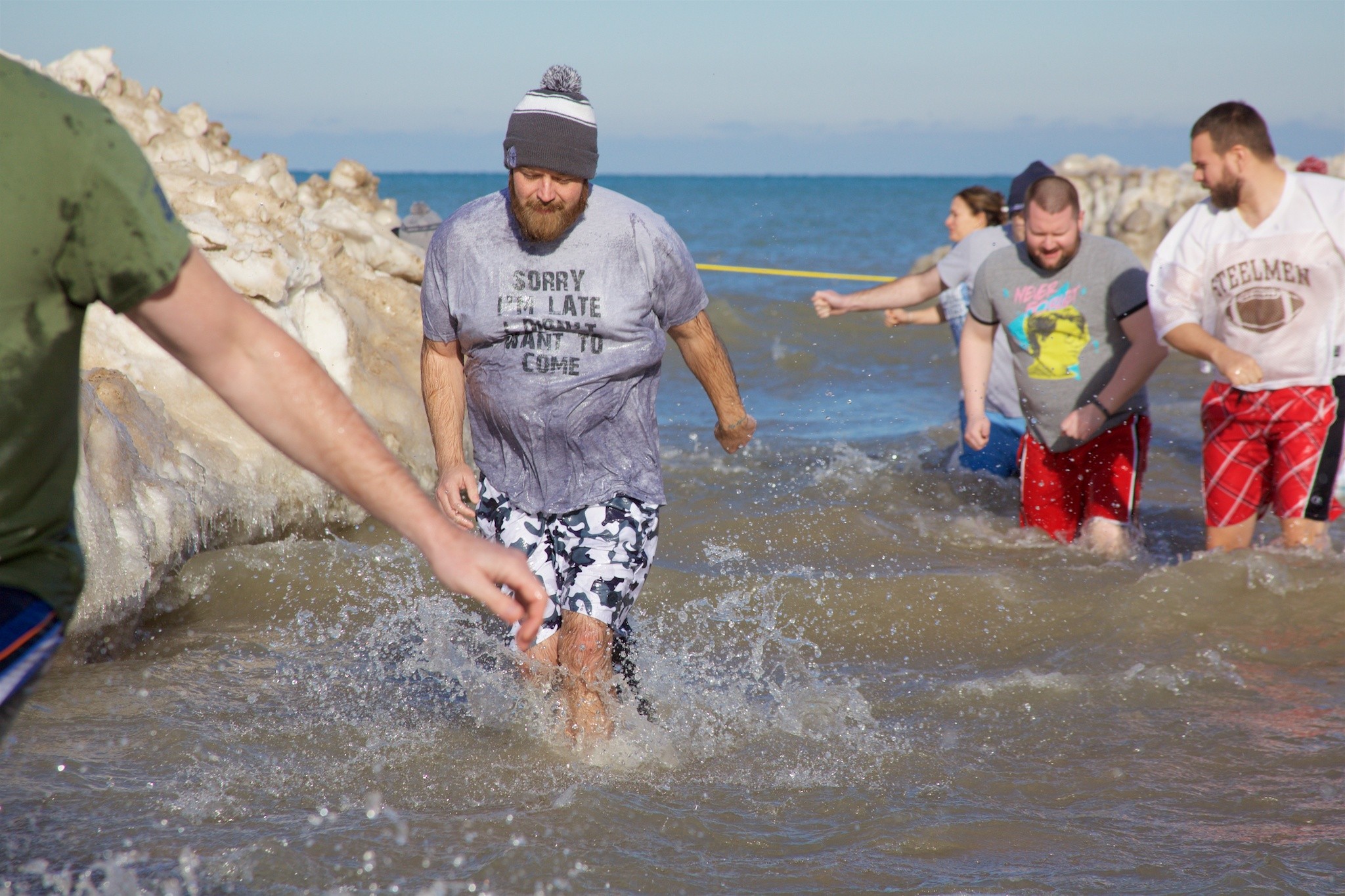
1007 160 1056 219
502 63 600 181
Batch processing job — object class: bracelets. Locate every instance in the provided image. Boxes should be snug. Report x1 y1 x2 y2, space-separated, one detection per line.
1089 398 1111 421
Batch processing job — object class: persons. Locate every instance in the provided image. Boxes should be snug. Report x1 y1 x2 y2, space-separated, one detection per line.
959 176 1167 542
419 62 756 739
885 186 1007 356
1145 100 1345 553
0 51 549 734
812 158 1054 477
